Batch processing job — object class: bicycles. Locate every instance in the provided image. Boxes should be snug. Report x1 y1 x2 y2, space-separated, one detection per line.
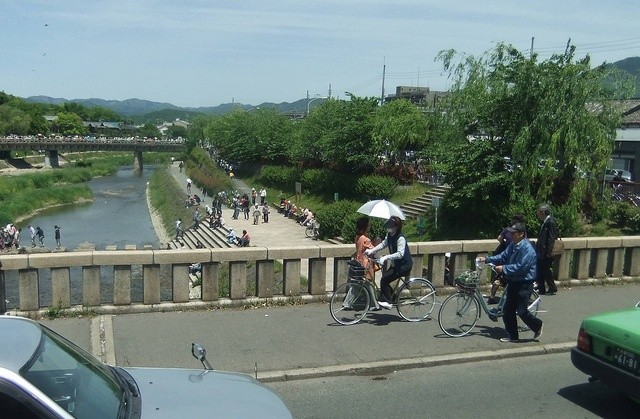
330 253 442 326
438 256 548 338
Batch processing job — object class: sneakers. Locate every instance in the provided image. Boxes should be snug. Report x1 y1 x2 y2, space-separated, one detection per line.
377 300 394 310
500 337 520 343
533 318 544 341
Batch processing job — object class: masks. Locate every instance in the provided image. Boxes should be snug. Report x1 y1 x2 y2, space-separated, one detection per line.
387 229 393 233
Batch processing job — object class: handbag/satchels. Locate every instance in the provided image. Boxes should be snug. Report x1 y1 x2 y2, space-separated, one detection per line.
546 219 564 257
351 235 362 262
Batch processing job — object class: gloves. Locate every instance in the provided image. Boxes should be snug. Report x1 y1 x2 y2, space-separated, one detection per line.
377 257 387 265
365 248 375 255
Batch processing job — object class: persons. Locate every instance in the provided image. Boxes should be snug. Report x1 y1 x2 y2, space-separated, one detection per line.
175 218 183 239
250 188 257 205
478 223 544 343
179 161 184 172
364 216 414 310
277 197 324 240
27 226 36 247
0 223 22 250
186 179 192 195
36 227 44 247
244 197 250 220
191 195 201 206
241 196 246 207
341 215 382 310
231 195 242 219
196 241 205 249
199 139 236 179
54 225 61 249
202 186 206 201
205 197 226 229
236 230 250 246
252 203 261 225
185 196 194 208
259 187 266 203
244 193 248 201
535 203 558 295
261 202 270 223
488 215 528 305
193 207 201 229
378 153 440 184
170 155 175 165
225 228 236 243
0 133 189 146
612 171 626 182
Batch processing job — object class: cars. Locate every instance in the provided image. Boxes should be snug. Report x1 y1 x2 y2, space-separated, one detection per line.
602 169 631 186
0 314 292 419
570 298 640 408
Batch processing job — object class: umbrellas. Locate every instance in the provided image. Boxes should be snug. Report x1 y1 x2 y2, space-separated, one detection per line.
187 179 192 183
356 199 407 220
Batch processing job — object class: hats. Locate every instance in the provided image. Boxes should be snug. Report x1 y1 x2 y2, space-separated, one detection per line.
382 216 403 228
252 188 255 190
507 222 526 233
